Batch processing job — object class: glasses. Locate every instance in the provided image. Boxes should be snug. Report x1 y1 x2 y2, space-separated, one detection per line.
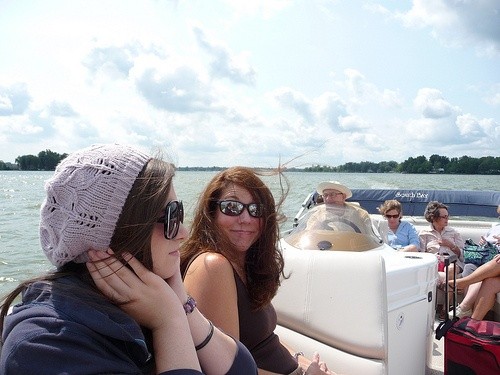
439 215 449 220
154 199 191 239
214 201 264 218
322 192 344 199
384 214 401 218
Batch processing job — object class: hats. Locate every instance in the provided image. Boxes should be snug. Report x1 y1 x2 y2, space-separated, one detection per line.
316 181 353 200
38 145 151 270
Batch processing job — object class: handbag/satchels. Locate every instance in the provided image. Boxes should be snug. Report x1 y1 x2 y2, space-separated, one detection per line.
464 238 499 265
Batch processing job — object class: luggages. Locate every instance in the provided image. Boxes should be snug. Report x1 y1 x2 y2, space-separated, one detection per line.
435 254 500 375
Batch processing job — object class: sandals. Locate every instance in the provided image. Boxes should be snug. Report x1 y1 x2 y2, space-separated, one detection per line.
437 282 463 295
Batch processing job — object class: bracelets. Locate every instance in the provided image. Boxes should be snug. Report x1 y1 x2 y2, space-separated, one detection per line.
183 294 197 315
195 320 214 351
295 351 304 364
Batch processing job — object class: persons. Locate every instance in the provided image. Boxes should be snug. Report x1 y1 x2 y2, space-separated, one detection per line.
304 180 500 321
0 144 258 375
179 166 331 375
